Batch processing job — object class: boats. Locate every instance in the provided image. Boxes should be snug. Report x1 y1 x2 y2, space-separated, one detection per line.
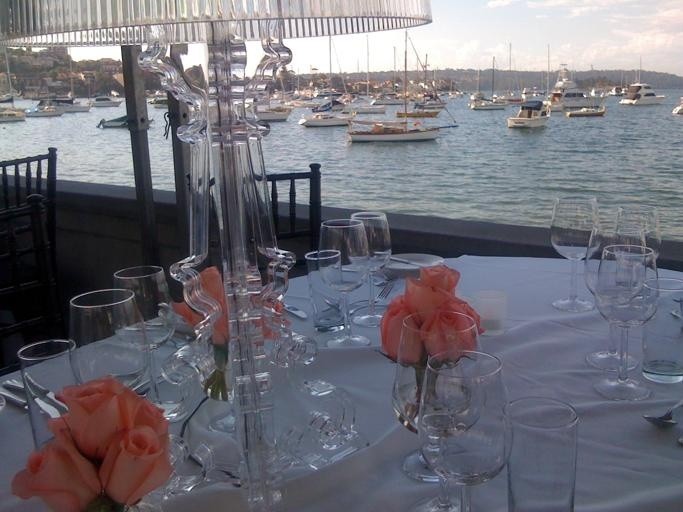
26 101 65 117
470 56 509 110
96 115 153 128
566 107 606 117
298 112 356 128
345 28 459 142
0 110 25 123
89 97 122 107
342 33 385 114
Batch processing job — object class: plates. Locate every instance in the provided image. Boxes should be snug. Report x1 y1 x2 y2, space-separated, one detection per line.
388 251 444 273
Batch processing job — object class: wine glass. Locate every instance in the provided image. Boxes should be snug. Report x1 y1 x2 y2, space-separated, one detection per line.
392 310 483 486
593 243 656 403
318 218 371 351
113 264 176 382
586 226 649 374
349 210 392 329
68 287 150 392
617 202 662 284
549 195 599 313
416 346 514 512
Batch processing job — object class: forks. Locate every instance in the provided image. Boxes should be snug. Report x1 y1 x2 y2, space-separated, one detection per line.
350 283 395 310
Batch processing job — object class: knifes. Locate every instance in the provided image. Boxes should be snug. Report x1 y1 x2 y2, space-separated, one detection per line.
2 371 68 418
392 256 427 268
286 300 308 321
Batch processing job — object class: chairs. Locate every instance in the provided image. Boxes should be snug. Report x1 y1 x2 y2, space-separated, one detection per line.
0 145 68 323
186 163 322 279
1 196 61 372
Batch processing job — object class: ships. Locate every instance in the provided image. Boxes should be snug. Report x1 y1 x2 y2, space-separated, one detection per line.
507 100 552 127
548 64 605 112
395 53 445 118
619 55 666 105
0 98 90 113
256 107 294 122
154 92 168 108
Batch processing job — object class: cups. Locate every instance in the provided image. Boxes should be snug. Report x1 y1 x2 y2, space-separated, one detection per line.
16 338 79 452
641 278 683 384
477 288 508 337
502 393 581 512
305 250 346 334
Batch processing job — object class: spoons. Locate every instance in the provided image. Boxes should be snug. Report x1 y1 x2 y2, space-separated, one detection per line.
644 395 682 426
